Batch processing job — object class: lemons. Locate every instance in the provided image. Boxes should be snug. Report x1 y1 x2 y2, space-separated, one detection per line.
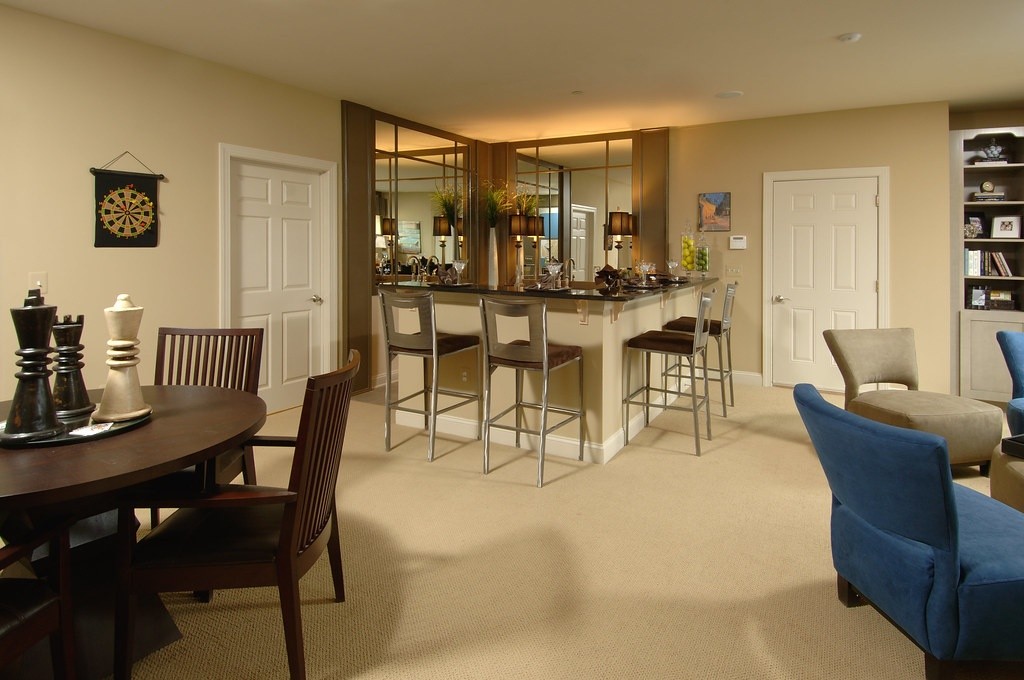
681 235 708 271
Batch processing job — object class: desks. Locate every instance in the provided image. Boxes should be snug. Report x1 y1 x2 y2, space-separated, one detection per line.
988 443 1024 515
377 273 719 466
0 384 267 680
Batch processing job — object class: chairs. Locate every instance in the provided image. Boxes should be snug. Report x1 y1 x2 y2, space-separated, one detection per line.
996 331 1024 436
0 517 80 680
134 328 360 680
823 327 1004 479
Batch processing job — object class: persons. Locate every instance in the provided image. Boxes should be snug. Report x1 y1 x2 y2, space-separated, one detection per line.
1000 221 1012 231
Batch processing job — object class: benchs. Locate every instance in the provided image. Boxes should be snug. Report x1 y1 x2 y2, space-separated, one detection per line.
793 383 1024 680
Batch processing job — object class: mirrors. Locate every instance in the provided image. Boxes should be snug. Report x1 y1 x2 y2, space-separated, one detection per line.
428 175 467 259
374 153 464 276
516 159 562 276
515 138 633 282
374 119 472 281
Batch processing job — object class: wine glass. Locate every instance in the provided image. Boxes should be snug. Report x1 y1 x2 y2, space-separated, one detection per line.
639 261 679 287
451 260 469 285
544 262 564 290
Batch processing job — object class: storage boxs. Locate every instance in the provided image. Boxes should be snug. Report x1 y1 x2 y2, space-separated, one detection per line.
1002 433 1024 459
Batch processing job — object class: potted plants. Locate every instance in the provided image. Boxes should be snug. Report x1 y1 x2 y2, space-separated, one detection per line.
516 186 542 279
469 176 519 287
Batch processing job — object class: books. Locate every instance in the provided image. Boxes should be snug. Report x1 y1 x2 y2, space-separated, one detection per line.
964 248 1012 277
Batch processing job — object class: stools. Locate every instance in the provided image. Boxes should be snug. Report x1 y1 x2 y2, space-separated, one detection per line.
664 280 739 417
478 296 585 487
378 288 483 461
621 288 718 456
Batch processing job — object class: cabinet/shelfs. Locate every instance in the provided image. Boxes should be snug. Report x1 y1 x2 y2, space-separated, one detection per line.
949 127 1024 413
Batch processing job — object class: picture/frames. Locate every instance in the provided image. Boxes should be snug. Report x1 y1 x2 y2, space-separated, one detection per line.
990 216 1021 238
964 211 990 238
965 285 991 310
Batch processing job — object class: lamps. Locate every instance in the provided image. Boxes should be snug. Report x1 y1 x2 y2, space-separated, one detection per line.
505 215 529 286
381 218 399 246
433 216 452 271
527 216 545 279
624 214 632 271
607 212 632 292
457 218 463 247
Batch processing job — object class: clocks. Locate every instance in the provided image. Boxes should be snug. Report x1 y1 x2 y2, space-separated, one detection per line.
981 180 995 193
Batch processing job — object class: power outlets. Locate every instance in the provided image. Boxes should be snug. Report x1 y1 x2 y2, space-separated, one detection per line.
458 367 471 384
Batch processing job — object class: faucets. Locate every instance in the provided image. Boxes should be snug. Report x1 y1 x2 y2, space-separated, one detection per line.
565 258 576 288
406 256 421 282
426 256 439 275
